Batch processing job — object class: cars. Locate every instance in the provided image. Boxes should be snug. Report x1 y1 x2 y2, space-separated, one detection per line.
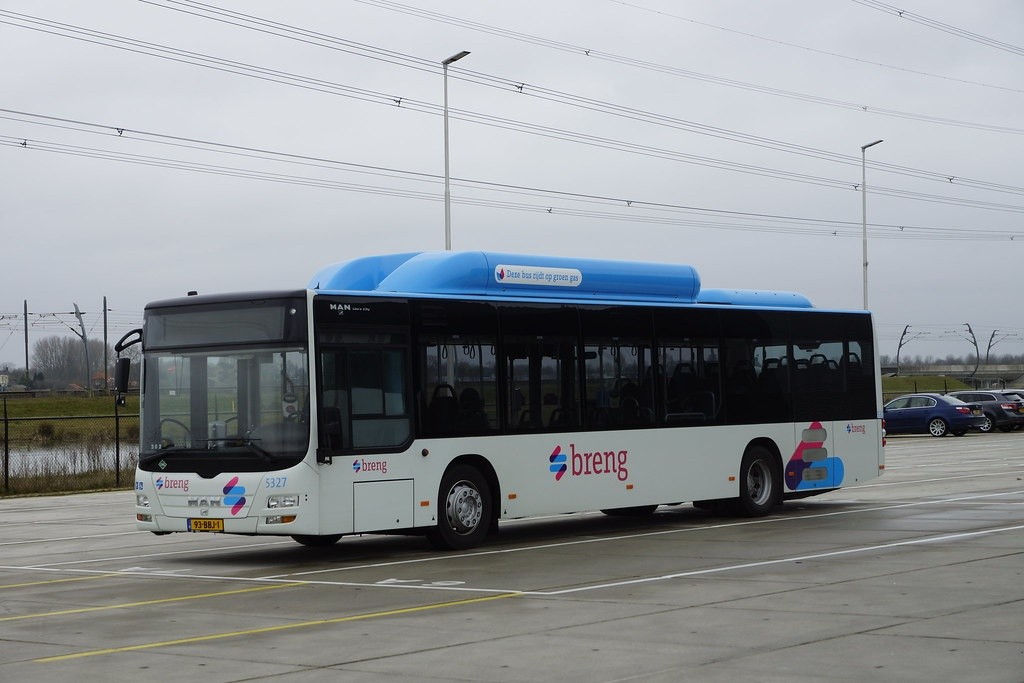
947 389 1024 433
884 392 975 437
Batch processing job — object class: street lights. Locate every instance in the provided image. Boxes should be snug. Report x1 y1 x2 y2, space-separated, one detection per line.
442 50 472 252
861 139 885 309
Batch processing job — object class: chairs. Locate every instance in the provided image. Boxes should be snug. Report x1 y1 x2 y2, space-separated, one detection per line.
426 351 861 436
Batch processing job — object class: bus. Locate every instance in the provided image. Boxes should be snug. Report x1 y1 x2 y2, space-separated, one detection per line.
113 249 890 556
